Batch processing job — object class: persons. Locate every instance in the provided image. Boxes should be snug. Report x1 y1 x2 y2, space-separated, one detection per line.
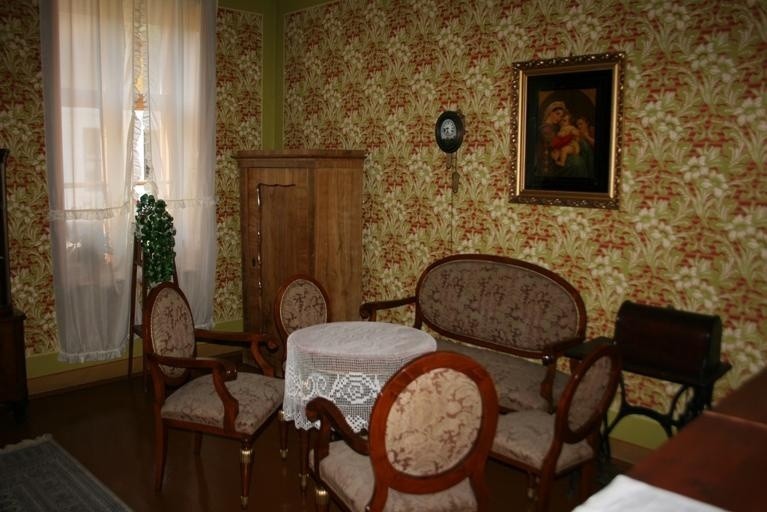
536 100 598 177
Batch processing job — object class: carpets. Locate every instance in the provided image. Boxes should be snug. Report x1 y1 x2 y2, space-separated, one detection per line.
0 431 135 512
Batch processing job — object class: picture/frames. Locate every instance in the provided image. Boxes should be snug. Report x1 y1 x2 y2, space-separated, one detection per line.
509 51 627 208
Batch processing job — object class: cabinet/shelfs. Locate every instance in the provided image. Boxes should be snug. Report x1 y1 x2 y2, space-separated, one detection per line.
0 146 31 430
231 146 366 367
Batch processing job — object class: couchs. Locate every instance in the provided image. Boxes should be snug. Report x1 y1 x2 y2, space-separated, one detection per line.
358 253 588 499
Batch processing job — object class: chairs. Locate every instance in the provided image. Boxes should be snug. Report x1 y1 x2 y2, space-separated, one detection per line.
565 299 733 439
142 281 288 511
306 349 500 512
486 340 623 511
273 274 332 378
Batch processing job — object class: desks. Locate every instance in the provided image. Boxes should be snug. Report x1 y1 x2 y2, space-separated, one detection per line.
704 360 767 429
573 411 767 512
280 321 436 492
